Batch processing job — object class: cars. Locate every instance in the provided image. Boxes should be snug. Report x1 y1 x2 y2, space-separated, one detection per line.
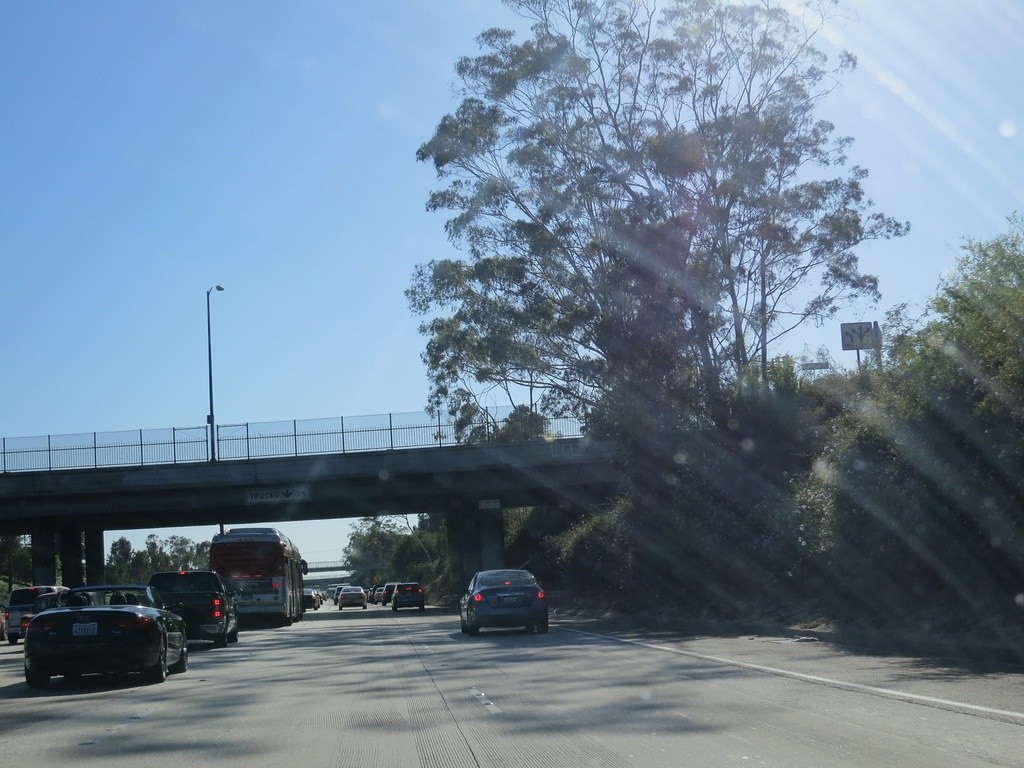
460 568 550 635
380 582 405 606
0 605 8 641
301 579 385 612
392 582 425 612
20 583 189 687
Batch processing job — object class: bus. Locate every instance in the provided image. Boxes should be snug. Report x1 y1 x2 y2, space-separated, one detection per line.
210 528 309 628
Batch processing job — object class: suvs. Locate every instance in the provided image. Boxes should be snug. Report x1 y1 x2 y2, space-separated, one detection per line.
140 569 243 647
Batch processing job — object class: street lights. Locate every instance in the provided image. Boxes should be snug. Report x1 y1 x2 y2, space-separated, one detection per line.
204 284 225 461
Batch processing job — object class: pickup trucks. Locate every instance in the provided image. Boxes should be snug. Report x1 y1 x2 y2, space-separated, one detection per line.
20 590 98 636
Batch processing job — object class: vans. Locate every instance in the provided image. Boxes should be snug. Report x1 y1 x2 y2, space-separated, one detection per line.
5 585 72 645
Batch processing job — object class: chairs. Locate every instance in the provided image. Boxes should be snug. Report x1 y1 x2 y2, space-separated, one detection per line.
109 595 127 606
67 595 88 608
125 594 139 604
